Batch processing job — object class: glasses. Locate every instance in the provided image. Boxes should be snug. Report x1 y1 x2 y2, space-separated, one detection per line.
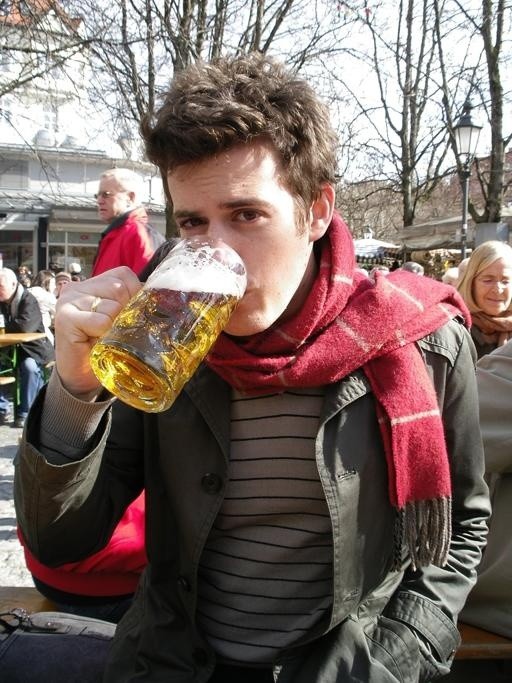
95 191 117 199
478 278 509 288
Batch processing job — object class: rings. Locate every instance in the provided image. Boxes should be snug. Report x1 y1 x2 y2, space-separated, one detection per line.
91 297 102 311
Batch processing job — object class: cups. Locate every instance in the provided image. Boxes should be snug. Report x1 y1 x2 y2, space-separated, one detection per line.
88 234 247 414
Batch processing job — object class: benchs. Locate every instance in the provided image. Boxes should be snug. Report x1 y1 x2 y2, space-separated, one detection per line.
456 621 512 659
0 361 55 386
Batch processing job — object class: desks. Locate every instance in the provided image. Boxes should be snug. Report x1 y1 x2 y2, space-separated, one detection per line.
0 333 48 346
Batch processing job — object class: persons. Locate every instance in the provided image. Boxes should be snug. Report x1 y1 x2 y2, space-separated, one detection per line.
370 258 470 286
455 239 512 358
0 263 83 427
457 339 512 643
13 52 493 682
89 169 166 276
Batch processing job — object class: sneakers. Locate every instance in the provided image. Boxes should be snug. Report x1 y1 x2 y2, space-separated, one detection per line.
15 416 27 428
0 411 11 424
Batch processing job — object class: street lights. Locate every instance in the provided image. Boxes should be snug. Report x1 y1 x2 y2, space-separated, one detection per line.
451 98 481 263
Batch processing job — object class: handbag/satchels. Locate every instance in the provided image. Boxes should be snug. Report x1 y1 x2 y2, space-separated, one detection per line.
0 609 116 683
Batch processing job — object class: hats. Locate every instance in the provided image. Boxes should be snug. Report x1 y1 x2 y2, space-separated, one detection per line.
55 273 72 282
69 263 82 274
